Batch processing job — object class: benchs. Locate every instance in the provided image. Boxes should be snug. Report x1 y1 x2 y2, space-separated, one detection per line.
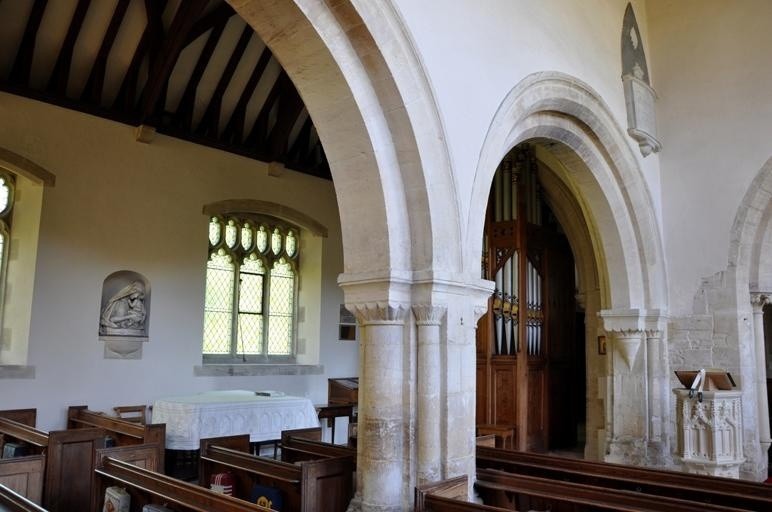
91 427 357 512
415 434 772 512
1 406 166 510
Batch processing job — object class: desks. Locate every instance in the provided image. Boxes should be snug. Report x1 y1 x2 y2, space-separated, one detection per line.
151 394 314 476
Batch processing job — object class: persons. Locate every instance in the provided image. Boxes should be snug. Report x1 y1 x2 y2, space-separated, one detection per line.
99 280 146 328
